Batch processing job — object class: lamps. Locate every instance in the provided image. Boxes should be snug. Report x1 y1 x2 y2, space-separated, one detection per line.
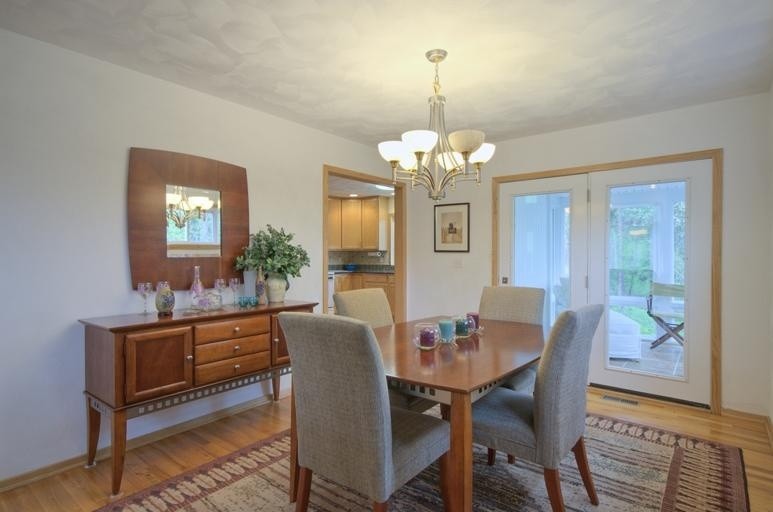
165 185 214 229
378 48 497 205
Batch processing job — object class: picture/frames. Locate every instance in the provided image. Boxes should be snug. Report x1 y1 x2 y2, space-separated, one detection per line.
433 203 470 253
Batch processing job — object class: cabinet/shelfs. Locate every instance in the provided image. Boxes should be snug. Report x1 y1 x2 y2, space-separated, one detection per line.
327 194 342 252
77 299 319 499
341 194 391 253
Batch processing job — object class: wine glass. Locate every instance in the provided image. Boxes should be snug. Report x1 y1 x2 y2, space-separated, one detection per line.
138 282 153 316
214 279 226 308
229 277 240 307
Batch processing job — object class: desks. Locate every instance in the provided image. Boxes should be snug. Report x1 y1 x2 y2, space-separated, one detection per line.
344 313 544 512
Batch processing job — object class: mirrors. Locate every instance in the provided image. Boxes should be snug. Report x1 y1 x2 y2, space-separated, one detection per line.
128 147 248 290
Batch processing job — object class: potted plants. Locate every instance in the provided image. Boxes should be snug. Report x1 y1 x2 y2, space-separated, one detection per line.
234 224 308 304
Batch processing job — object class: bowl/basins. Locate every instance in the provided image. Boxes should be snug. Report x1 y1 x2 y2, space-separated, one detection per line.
344 264 358 271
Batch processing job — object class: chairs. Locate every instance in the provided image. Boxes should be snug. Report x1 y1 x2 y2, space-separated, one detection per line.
474 285 545 466
275 311 450 512
467 306 597 512
332 286 446 418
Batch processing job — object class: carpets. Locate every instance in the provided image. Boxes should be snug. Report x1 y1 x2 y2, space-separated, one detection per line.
92 406 751 512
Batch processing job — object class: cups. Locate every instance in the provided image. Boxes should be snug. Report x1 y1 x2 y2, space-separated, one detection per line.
238 296 248 306
250 297 258 306
